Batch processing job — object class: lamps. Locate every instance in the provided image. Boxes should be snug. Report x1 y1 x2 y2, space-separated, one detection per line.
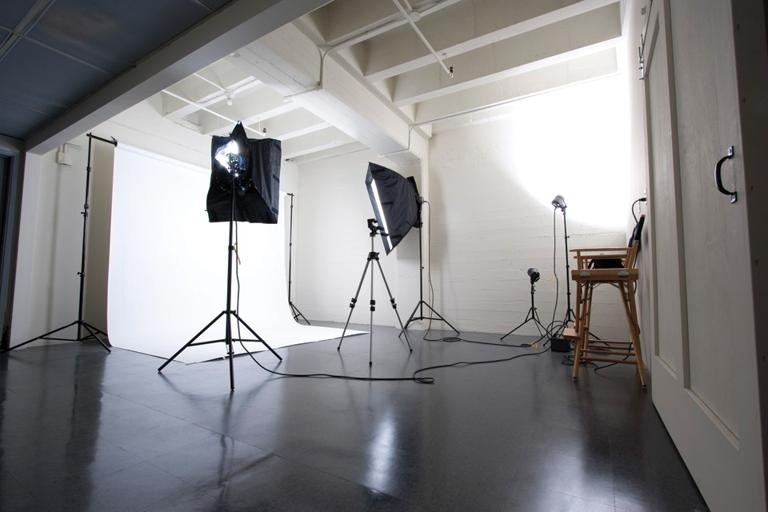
363 161 461 337
156 120 282 392
500 268 553 343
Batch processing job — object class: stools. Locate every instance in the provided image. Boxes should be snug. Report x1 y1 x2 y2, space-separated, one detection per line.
569 239 647 387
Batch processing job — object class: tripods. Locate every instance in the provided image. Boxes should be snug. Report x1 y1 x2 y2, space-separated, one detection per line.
500 281 553 344
337 232 413 367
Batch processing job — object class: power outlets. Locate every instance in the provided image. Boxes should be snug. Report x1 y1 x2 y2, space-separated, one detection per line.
643 187 648 205
